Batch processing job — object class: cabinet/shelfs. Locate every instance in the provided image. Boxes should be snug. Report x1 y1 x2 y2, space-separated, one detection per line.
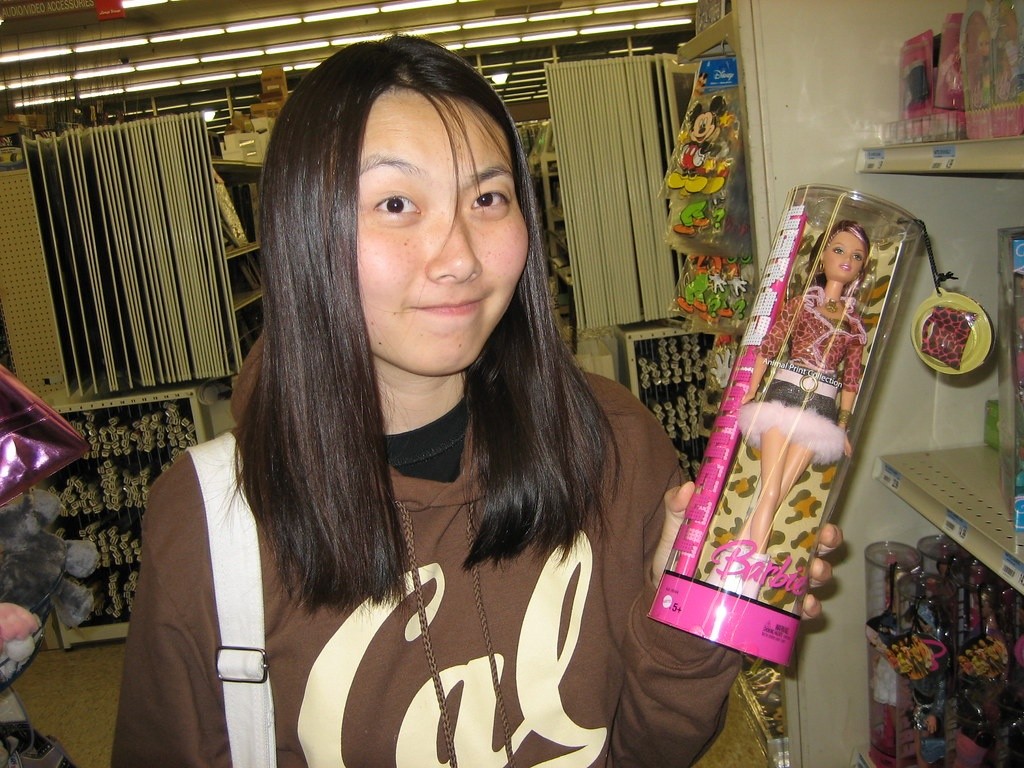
848 133 1024 768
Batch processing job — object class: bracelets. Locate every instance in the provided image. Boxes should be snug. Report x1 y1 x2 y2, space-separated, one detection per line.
838 410 850 423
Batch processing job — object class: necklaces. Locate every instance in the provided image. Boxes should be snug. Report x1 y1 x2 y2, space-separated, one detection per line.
826 299 839 312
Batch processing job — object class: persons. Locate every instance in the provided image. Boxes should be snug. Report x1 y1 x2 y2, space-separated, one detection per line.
866 552 1024 768
705 220 871 599
111 35 843 768
